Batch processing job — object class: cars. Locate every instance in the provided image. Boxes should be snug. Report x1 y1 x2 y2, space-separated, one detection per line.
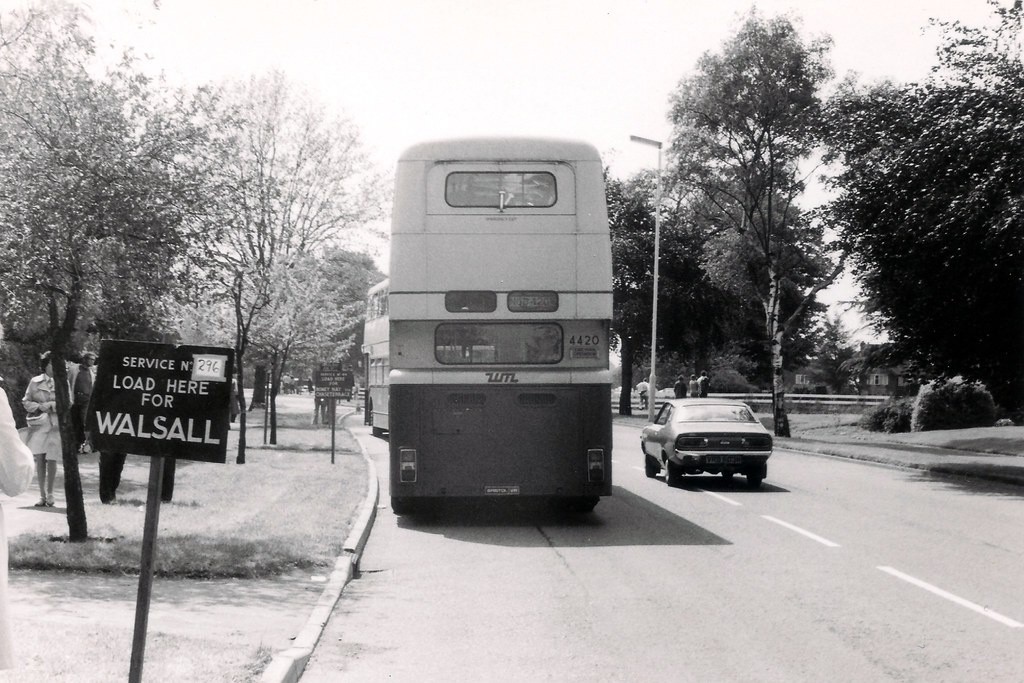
640 397 773 487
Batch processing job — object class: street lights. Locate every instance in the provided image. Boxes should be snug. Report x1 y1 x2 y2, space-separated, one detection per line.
628 134 663 424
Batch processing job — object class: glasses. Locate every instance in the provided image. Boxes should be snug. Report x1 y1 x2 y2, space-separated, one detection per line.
86 357 96 362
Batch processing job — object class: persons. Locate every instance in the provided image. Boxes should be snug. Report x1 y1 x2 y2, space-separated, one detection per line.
282 372 295 394
674 375 687 399
312 397 327 424
697 370 710 398
22 351 73 507
352 384 358 401
68 353 98 454
637 378 649 411
84 375 127 504
308 378 314 394
690 374 698 398
297 375 304 394
0 373 34 671
227 375 238 430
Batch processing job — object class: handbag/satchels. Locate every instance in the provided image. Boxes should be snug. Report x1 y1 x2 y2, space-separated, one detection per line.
25 412 51 432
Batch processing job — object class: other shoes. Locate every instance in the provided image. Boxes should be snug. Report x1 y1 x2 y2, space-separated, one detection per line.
35 497 47 506
47 495 54 507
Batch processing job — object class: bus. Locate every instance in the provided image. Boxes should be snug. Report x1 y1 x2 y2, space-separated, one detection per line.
357 277 389 438
388 139 614 520
